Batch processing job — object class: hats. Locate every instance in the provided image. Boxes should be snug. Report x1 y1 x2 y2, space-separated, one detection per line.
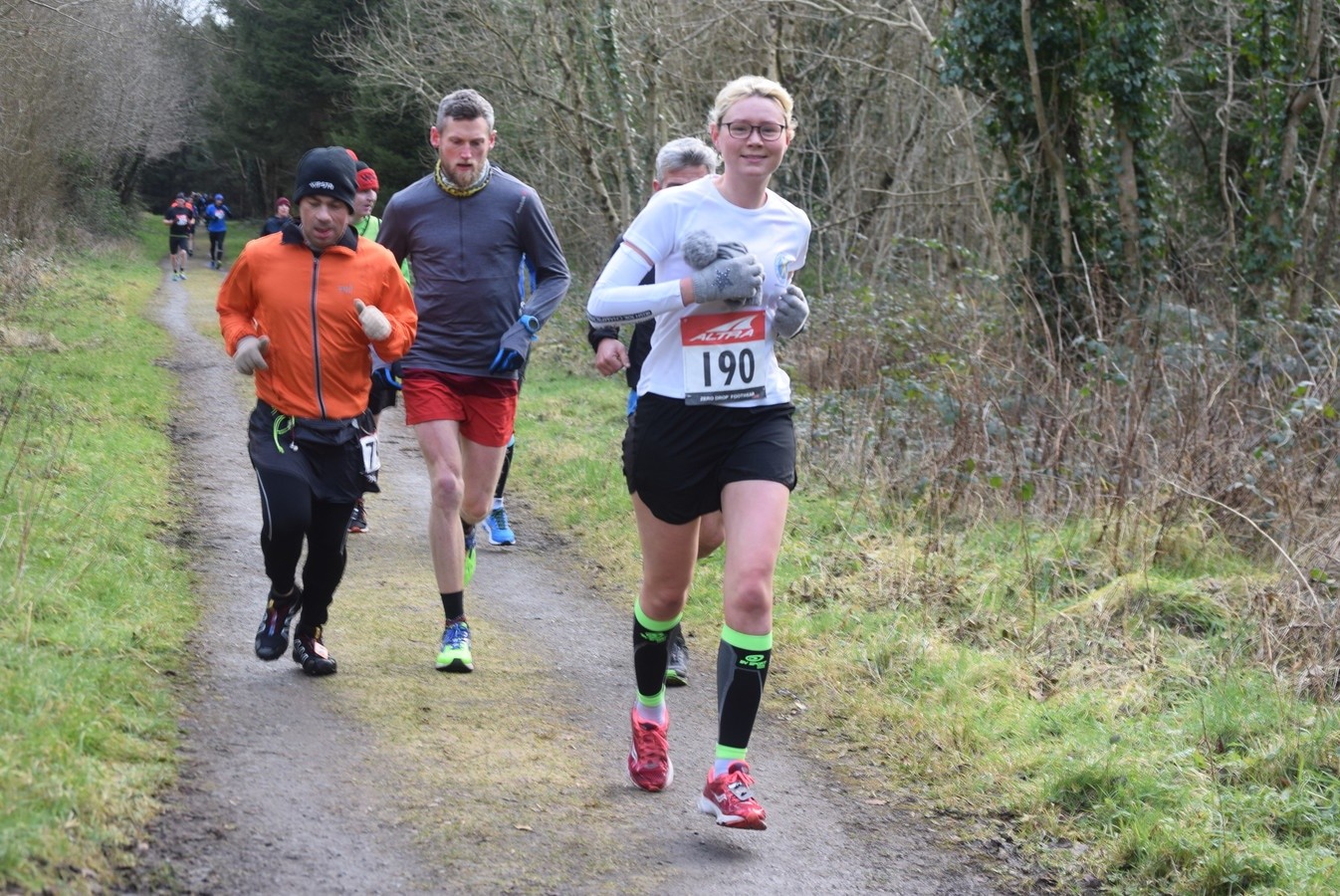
294 146 357 215
683 230 763 310
275 197 291 209
346 149 379 194
215 193 223 200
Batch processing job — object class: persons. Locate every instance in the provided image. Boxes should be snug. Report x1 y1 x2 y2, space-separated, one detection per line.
586 74 811 830
204 194 231 271
262 196 294 239
216 86 572 677
346 149 413 532
163 190 214 281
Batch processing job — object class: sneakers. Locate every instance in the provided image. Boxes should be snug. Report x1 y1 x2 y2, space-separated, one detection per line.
465 528 477 587
628 699 673 792
435 622 473 672
254 583 302 662
664 641 690 688
346 499 368 532
293 622 337 677
699 759 768 830
482 507 516 545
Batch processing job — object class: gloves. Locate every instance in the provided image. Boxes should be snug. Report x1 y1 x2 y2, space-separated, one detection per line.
773 285 811 340
232 334 269 375
489 349 524 375
371 367 403 394
691 254 762 305
353 297 392 341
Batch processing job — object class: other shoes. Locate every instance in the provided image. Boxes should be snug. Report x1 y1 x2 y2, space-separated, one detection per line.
173 274 178 281
211 260 215 268
217 260 222 267
179 272 186 280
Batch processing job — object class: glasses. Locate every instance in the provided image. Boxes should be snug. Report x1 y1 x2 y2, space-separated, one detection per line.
719 121 787 141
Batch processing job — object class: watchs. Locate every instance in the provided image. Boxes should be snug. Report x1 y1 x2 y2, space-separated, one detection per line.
521 315 540 334
